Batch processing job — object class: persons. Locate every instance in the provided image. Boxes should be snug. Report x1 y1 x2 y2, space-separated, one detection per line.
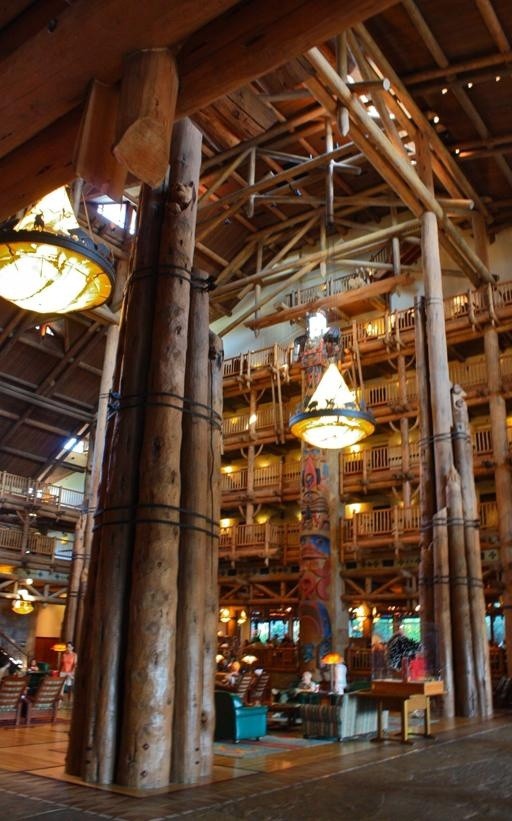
30 659 40 671
387 630 426 719
215 629 301 690
57 641 78 711
292 671 317 725
14 686 34 728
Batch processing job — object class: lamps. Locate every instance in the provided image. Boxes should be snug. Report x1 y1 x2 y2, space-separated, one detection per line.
321 652 344 691
0 177 116 314
11 599 34 615
289 326 377 449
50 643 66 670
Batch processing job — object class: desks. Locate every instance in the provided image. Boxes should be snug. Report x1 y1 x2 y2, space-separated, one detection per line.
348 677 448 745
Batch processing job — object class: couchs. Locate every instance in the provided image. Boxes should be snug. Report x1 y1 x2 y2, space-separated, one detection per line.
214 691 271 743
298 689 390 742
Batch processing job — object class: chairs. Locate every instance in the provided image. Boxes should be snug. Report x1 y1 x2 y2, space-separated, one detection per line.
22 676 68 724
215 672 254 698
0 676 30 725
246 671 271 703
